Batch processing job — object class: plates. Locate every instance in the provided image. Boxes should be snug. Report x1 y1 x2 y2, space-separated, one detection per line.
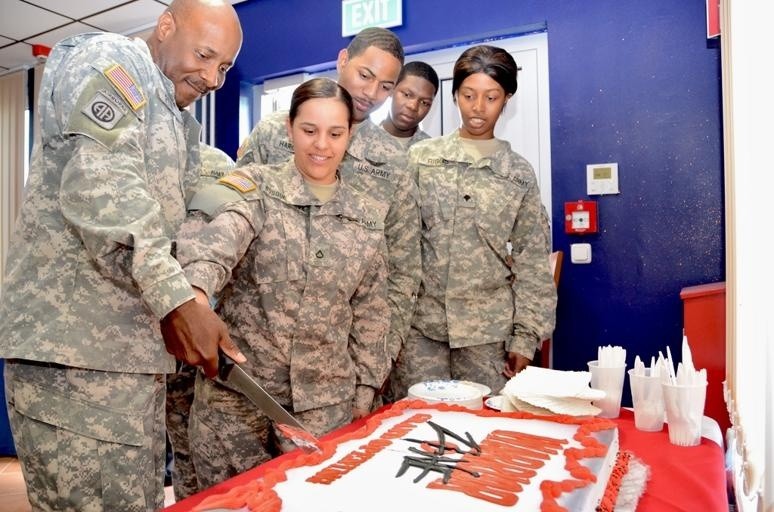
406 379 491 410
483 395 503 411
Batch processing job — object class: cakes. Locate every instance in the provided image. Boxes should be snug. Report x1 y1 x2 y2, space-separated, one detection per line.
190 401 646 512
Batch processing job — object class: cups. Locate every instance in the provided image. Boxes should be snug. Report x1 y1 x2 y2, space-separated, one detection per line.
627 369 671 432
662 381 708 446
587 362 628 419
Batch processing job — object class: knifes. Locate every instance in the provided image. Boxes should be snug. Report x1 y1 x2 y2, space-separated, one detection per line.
218 348 321 455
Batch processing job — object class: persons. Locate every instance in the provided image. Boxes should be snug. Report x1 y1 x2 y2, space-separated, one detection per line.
392 46 557 402
177 78 390 492
164 139 237 502
234 28 422 423
0 0 246 512
372 62 438 149
